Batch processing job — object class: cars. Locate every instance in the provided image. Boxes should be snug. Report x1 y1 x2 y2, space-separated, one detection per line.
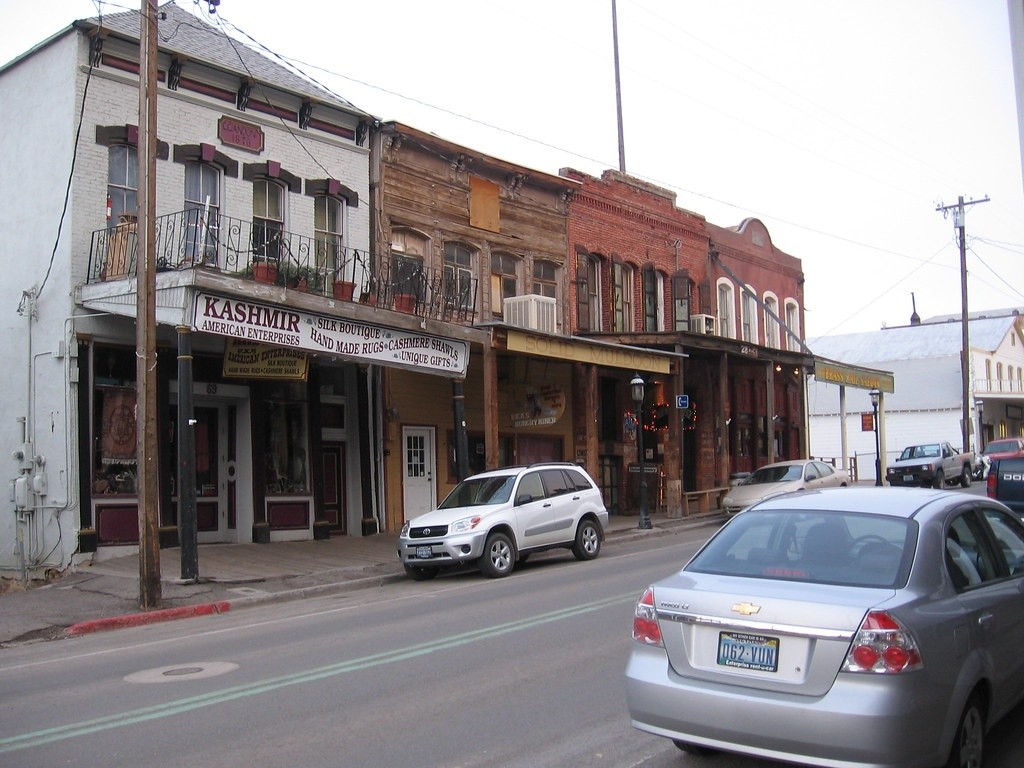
723 458 850 522
979 437 1024 466
622 488 1024 768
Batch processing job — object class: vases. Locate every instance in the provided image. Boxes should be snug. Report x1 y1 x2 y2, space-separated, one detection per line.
395 293 417 314
331 281 357 301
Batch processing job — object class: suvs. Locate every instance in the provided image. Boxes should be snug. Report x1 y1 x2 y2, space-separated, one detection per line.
396 462 609 581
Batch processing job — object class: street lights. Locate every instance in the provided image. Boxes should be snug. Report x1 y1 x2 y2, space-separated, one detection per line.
868 385 883 487
630 371 653 529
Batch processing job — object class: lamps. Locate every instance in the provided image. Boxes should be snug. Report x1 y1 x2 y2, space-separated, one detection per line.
794 368 799 375
775 364 781 372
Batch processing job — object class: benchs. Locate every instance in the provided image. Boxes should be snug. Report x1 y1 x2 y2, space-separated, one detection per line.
681 486 729 517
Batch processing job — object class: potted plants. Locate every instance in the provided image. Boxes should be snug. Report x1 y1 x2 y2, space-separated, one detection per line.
253 261 278 285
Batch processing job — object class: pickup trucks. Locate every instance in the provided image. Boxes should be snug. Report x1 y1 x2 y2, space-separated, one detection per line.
985 457 1024 512
885 441 975 490
